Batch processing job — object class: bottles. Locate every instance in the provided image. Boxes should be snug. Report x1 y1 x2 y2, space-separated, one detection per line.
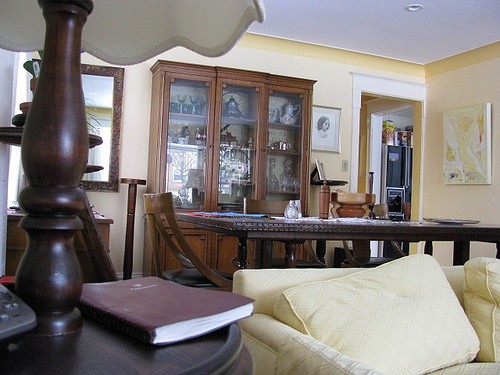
267 158 278 191
166 121 202 145
283 201 298 219
280 157 298 192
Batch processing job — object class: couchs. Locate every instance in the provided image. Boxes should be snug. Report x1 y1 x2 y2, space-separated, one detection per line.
233 266 500 375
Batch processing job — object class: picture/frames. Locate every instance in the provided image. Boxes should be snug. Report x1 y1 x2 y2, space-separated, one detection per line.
312 105 342 153
442 104 491 184
79 64 125 192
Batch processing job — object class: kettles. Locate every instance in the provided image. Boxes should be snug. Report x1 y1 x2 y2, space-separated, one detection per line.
222 89 244 118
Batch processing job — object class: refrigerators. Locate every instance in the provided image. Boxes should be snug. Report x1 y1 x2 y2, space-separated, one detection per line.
377 142 413 258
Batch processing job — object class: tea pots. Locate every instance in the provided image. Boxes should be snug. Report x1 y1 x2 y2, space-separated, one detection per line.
268 108 282 123
276 100 302 125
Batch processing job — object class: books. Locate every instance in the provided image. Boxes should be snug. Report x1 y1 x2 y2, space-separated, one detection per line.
75 275 255 347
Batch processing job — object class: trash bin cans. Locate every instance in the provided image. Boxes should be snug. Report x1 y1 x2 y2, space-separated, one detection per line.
334 247 371 268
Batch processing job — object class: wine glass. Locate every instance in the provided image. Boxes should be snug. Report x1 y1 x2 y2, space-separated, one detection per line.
198 94 208 116
176 93 187 113
189 94 200 115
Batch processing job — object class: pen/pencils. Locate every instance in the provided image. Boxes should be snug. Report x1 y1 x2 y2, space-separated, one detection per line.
263 217 275 220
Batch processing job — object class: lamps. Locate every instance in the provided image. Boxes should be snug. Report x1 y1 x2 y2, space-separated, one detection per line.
0 0 264 337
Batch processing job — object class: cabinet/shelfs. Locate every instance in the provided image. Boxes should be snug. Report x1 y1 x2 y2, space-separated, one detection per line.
143 59 317 273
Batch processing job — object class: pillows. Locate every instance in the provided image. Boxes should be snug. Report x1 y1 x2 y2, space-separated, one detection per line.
273 253 480 374
463 257 499 363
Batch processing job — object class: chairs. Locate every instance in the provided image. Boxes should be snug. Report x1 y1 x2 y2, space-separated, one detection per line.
244 197 325 268
144 192 233 290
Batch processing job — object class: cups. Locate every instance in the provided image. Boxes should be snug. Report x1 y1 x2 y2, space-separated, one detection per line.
168 135 173 142
169 102 178 112
268 138 293 150
177 137 188 144
182 103 200 115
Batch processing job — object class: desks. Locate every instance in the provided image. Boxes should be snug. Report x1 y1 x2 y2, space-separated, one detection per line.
176 212 500 269
5 207 113 277
0 321 254 375
